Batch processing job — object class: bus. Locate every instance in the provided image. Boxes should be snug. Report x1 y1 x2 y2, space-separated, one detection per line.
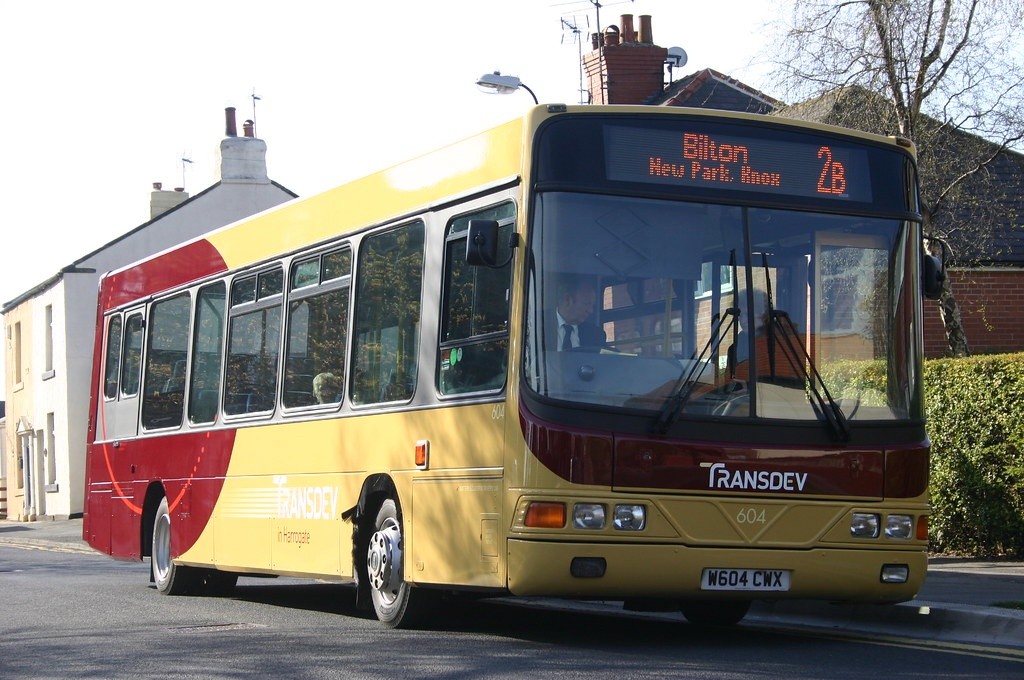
80 69 949 633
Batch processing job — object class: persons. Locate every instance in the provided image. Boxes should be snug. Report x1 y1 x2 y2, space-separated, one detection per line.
312 371 340 404
541 272 618 352
727 291 793 380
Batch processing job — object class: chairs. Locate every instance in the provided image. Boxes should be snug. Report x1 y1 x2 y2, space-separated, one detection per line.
314 371 342 403
162 360 188 391
127 357 138 395
194 390 216 423
286 373 313 395
379 368 410 402
284 391 312 408
106 369 118 398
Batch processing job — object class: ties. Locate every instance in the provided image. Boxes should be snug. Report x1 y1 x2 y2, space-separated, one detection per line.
561 323 575 351
